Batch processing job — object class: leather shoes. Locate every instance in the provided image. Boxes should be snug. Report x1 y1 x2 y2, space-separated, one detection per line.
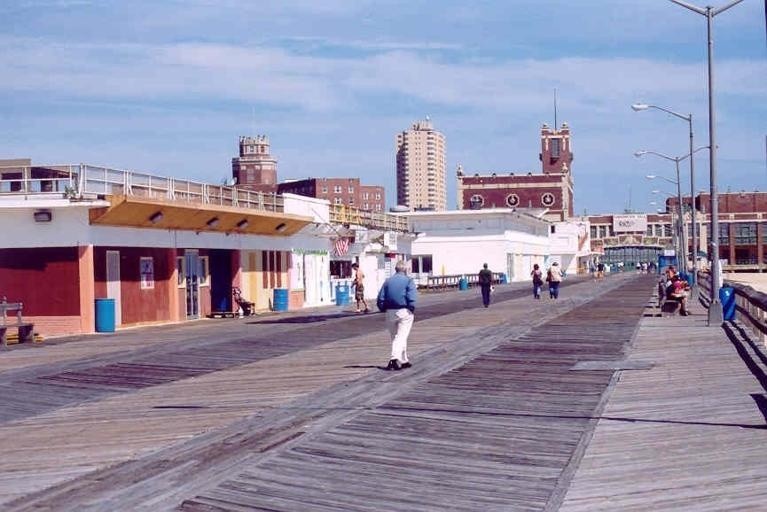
388 360 411 370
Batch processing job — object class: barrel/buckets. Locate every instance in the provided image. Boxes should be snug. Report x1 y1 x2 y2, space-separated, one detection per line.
500 274 507 284
96 299 116 333
687 273 694 282
336 285 349 305
720 286 738 320
459 278 467 290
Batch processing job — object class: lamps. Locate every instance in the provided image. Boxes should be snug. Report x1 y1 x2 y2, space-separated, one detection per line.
34 212 52 222
237 219 249 229
149 211 163 224
207 217 220 227
275 223 288 233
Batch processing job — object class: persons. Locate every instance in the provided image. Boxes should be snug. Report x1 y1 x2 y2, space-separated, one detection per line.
530 264 542 299
590 258 604 279
479 263 494 308
547 262 567 298
234 287 256 316
351 263 371 312
616 260 624 273
636 258 691 316
376 266 418 369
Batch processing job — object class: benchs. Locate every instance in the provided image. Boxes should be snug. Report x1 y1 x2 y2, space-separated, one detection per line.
659 282 680 316
0 303 34 346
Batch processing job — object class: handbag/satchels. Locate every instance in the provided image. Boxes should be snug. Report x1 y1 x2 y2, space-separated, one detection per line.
534 279 543 286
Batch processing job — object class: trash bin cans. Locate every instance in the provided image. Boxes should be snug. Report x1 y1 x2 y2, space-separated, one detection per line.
499 274 506 284
94 298 114 332
718 287 736 319
335 285 349 306
459 279 467 290
679 271 693 287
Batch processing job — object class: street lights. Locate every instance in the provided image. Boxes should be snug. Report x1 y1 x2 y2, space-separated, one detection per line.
630 103 719 301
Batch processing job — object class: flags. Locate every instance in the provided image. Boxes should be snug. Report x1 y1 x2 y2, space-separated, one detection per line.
332 237 351 257
